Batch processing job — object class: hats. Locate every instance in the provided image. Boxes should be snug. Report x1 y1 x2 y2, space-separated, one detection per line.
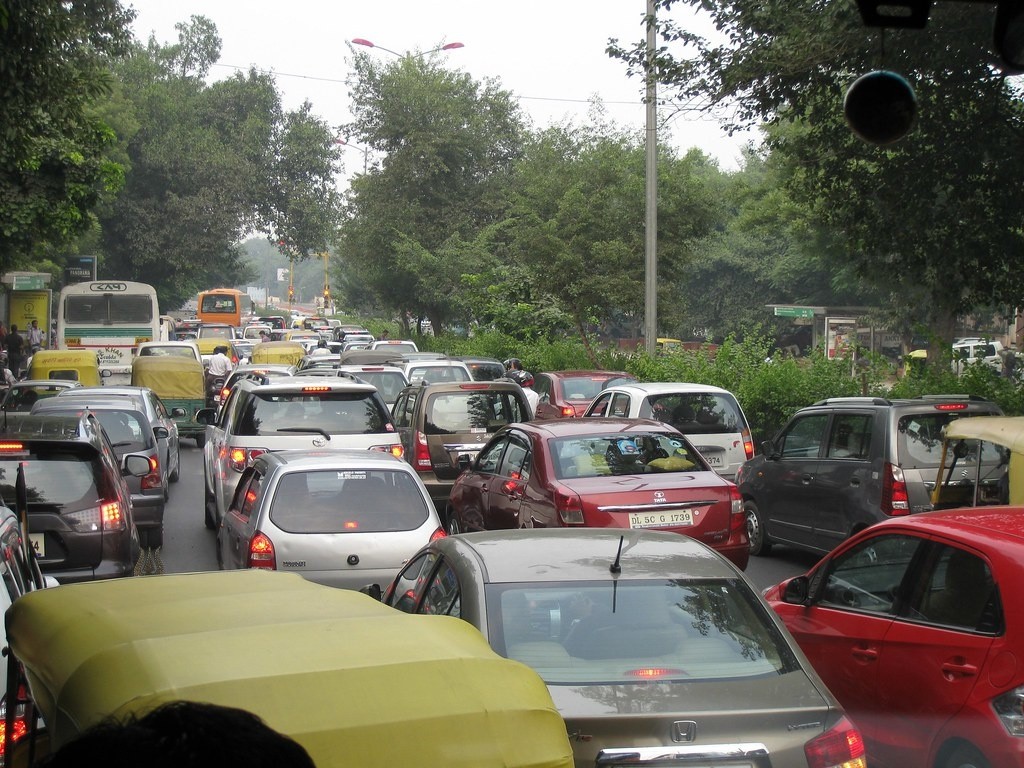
220 346 230 352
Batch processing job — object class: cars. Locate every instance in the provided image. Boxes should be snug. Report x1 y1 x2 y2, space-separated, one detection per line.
527 371 641 423
953 337 1010 376
26 347 102 391
135 357 206 449
450 419 751 575
363 528 869 767
212 450 444 594
26 386 186 500
156 314 531 449
761 506 1024 768
4 568 575 763
0 505 66 637
23 403 172 527
0 418 147 580
928 416 1024 510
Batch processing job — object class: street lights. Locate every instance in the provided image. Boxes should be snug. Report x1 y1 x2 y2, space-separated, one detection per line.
349 37 463 63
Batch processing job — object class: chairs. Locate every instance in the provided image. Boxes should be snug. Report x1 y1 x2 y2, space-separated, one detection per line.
337 476 388 516
925 554 989 623
837 432 868 460
34 458 79 503
278 475 312 517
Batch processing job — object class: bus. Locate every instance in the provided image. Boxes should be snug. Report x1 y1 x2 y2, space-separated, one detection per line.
198 287 251 330
57 281 163 383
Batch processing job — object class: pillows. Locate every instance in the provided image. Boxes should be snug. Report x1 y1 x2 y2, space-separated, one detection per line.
648 456 695 471
572 453 612 476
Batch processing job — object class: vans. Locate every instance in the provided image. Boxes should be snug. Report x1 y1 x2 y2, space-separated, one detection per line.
203 377 405 533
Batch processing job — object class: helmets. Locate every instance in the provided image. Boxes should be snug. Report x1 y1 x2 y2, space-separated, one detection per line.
510 370 534 387
318 340 328 348
339 331 346 337
0 353 8 367
503 359 512 371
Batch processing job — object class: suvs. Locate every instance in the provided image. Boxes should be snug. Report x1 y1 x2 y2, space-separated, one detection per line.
734 394 1013 557
581 380 757 476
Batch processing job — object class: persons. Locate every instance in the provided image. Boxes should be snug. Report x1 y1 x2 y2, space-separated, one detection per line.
832 424 866 457
0 320 56 404
376 329 389 341
205 346 232 390
335 331 345 343
175 318 182 328
311 340 332 356
494 357 540 423
259 331 270 343
35 700 316 768
